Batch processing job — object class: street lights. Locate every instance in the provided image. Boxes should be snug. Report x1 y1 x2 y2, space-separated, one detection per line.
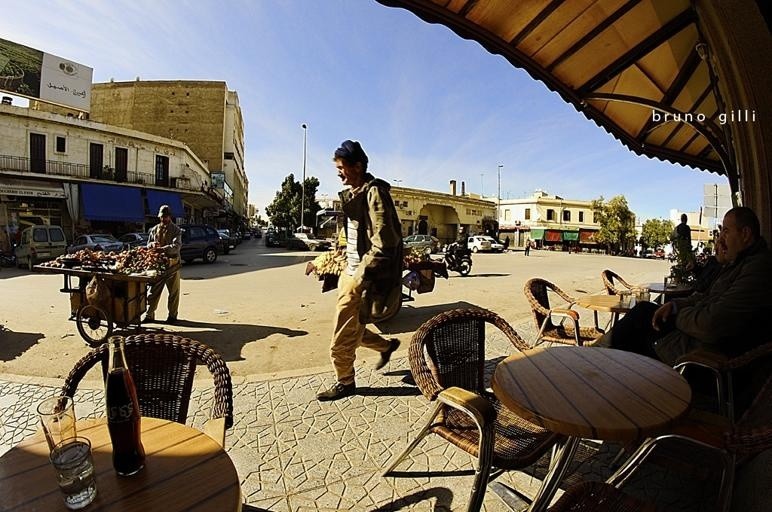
299 122 309 236
393 178 403 185
480 172 486 198
497 163 504 215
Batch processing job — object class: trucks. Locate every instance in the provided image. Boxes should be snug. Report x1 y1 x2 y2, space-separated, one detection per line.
476 234 504 252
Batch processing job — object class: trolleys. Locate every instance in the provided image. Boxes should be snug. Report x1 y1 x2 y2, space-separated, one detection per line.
29 244 190 349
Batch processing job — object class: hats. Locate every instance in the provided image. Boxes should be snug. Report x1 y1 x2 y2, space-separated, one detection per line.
158 206 172 218
335 140 368 163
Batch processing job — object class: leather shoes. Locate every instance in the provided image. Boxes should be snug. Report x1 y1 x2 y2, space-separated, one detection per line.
316 383 355 400
376 338 400 372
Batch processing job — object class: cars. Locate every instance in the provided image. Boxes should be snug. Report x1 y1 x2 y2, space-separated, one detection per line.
642 249 657 259
285 232 332 252
448 235 491 253
66 232 125 254
654 248 666 260
214 226 285 254
116 232 149 245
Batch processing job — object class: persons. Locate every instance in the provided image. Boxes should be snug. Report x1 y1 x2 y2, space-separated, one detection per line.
525 237 530 256
584 206 772 371
452 225 468 268
314 140 403 402
141 204 183 324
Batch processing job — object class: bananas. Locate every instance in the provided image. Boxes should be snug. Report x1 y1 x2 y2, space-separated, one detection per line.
315 249 348 276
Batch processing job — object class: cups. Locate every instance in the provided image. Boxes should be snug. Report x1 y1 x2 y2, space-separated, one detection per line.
45 435 103 511
37 394 79 449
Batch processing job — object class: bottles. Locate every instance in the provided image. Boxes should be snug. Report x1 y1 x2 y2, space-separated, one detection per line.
105 333 145 478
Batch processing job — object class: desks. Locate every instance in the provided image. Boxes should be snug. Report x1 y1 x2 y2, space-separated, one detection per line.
0 415 245 512
489 344 693 512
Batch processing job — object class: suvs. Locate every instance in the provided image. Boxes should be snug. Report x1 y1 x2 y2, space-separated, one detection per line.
401 233 442 255
133 224 224 267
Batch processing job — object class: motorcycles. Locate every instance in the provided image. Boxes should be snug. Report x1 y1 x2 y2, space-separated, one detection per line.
0 249 16 267
430 243 473 279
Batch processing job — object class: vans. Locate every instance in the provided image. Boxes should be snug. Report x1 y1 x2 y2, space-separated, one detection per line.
14 225 68 270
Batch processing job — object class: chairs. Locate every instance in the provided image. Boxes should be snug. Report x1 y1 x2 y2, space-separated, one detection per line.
520 268 771 512
380 305 564 512
51 332 235 452
